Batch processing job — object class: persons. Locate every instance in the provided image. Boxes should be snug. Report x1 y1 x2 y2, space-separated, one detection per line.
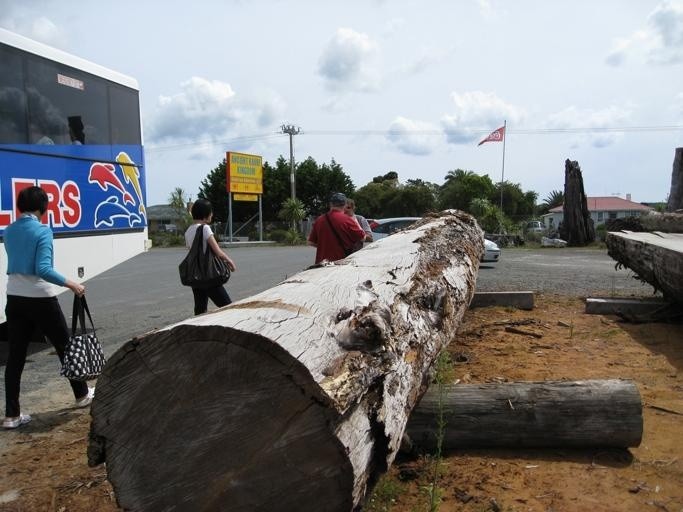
185 200 236 316
3 186 97 429
308 193 373 265
30 122 83 145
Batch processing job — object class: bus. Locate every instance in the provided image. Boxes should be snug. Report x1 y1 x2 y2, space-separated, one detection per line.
0 28 152 324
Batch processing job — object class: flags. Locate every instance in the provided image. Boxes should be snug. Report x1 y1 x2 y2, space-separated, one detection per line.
479 127 504 145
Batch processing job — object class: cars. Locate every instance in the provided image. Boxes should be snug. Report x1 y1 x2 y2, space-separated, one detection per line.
363 217 501 267
158 224 181 233
526 221 546 233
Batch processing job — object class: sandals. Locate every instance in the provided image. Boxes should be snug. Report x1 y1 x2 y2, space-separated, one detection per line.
2 413 32 429
74 386 96 408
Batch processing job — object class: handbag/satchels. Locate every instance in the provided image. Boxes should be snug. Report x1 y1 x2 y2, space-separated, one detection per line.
343 243 360 257
59 295 106 381
178 223 231 286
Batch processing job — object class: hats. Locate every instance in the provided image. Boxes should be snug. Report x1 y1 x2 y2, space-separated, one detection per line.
329 193 347 204
